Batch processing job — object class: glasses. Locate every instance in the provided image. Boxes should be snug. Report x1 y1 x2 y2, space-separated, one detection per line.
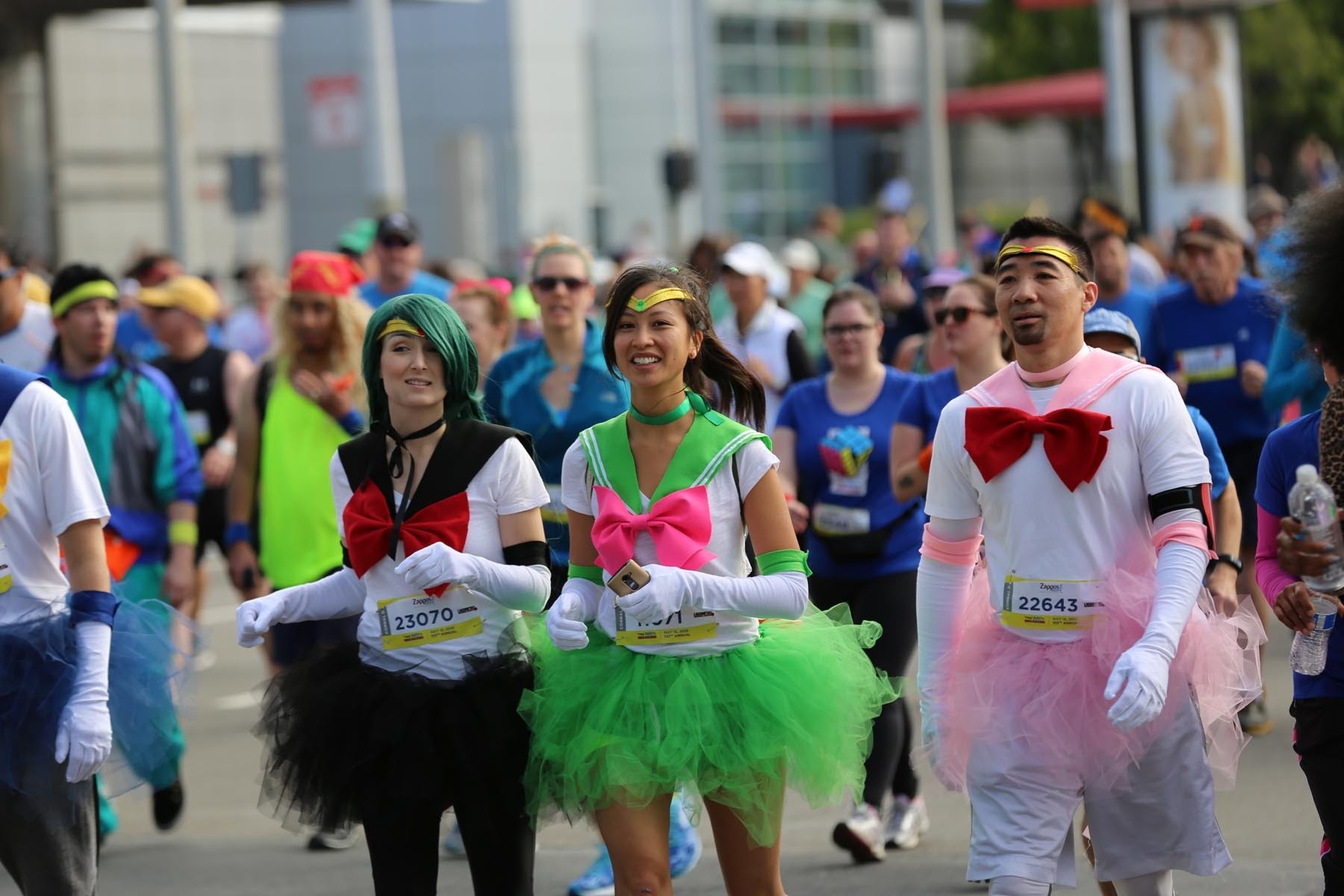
379 237 410 247
934 307 991 324
532 276 590 292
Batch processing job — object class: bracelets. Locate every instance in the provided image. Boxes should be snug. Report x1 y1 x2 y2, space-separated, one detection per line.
568 561 604 587
167 522 198 547
919 441 933 473
68 590 122 631
918 522 985 563
225 525 254 547
502 541 551 570
338 408 364 436
1151 521 1219 560
755 550 812 577
340 541 353 568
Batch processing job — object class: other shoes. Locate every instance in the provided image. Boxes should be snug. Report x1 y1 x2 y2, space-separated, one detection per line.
309 822 363 848
443 821 467 857
1237 688 1272 735
153 779 183 829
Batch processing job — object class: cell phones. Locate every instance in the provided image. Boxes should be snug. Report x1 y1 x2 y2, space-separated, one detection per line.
605 557 650 597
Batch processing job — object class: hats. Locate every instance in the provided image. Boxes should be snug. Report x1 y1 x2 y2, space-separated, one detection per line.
1084 307 1141 361
719 241 774 281
1177 218 1230 253
376 211 418 241
138 277 222 322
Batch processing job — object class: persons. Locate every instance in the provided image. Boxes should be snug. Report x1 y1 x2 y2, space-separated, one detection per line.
916 217 1269 896
236 294 554 896
0 186 1329 896
518 262 906 896
1254 409 1344 896
1272 179 1344 618
0 358 122 896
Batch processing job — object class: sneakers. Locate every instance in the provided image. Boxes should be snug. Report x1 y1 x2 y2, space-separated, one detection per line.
832 802 887 861
883 791 930 849
669 791 702 878
568 845 615 896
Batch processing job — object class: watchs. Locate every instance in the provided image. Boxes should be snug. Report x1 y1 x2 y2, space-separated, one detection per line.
1213 554 1243 575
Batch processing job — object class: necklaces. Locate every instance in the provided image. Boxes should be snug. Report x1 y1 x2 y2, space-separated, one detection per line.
630 391 724 425
385 415 446 561
1016 341 1089 383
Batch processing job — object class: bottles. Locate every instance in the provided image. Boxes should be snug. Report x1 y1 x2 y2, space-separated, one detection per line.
1288 464 1343 592
1290 592 1340 675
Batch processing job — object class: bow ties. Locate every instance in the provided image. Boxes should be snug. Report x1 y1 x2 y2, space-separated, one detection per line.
966 406 1113 495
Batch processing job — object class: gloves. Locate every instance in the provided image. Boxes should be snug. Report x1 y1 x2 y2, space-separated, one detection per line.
545 560 605 651
54 590 123 782
915 522 984 794
616 549 813 621
395 540 552 613
235 540 366 648
1102 521 1218 731
